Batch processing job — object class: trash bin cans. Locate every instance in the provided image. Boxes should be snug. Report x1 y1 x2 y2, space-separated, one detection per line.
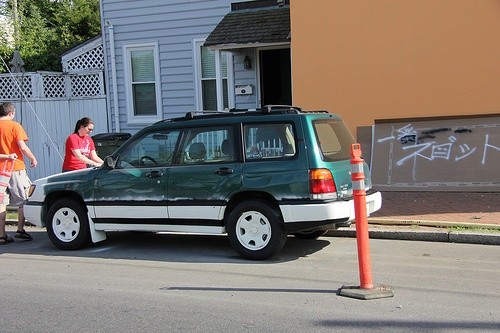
91 133 132 162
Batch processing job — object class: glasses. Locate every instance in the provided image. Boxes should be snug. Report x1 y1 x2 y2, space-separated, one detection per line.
84 126 94 132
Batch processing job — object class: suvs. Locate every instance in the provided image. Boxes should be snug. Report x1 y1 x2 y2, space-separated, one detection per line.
16 105 382 260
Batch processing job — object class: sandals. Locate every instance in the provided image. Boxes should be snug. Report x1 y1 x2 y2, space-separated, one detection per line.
0 235 15 245
14 229 33 240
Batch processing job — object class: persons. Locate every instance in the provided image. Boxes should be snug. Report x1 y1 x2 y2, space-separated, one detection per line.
0 102 38 245
62 117 104 172
